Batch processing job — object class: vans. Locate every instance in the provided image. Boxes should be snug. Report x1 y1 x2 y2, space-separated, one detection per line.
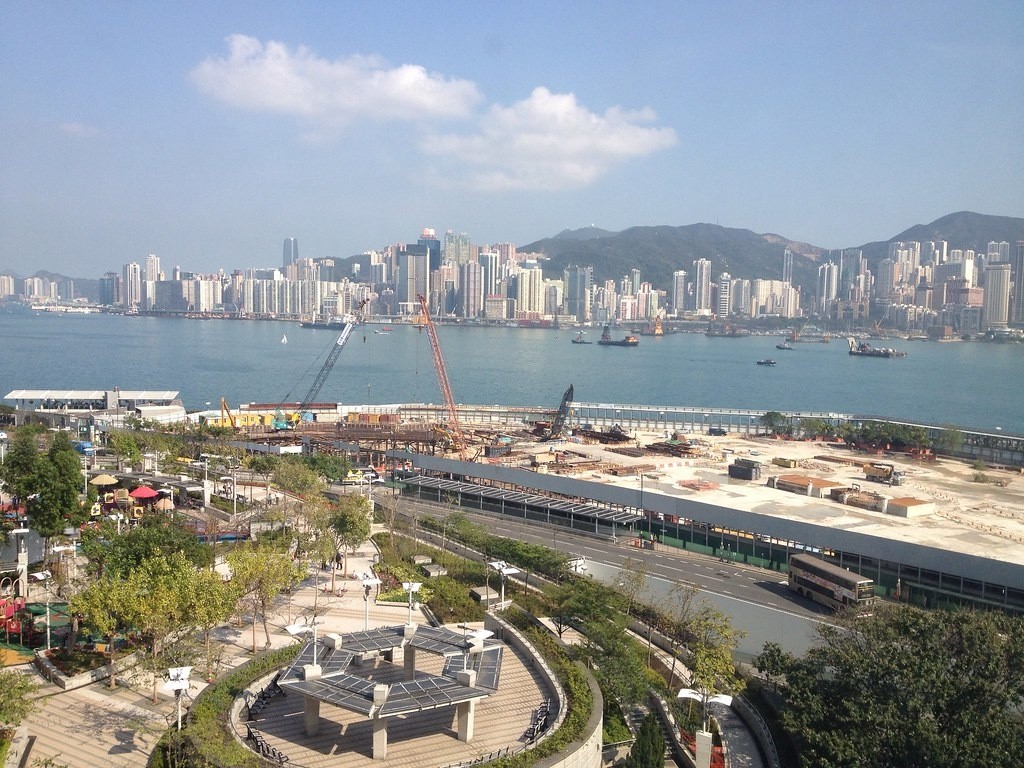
709 428 727 437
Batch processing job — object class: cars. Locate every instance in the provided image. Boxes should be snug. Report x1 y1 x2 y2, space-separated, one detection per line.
677 428 690 434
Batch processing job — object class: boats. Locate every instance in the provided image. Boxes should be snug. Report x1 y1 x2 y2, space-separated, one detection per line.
756 359 776 367
847 338 908 358
654 315 665 338
571 339 593 344
373 330 390 335
383 326 395 331
598 325 640 347
776 345 795 350
413 324 425 328
735 329 750 337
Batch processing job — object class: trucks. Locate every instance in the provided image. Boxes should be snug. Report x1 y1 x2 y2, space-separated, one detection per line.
67 440 94 456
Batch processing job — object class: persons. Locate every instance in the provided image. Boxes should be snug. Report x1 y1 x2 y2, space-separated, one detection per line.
320 553 343 571
719 550 732 563
649 531 658 546
351 569 372 580
223 480 233 498
766 554 781 571
265 491 280 506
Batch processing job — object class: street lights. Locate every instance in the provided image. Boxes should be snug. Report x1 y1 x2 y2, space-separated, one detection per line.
220 473 237 520
640 473 659 548
143 450 158 472
193 458 207 481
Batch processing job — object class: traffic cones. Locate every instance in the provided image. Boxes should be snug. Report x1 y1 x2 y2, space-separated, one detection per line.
324 583 328 593
343 583 347 592
337 588 343 597
351 570 357 579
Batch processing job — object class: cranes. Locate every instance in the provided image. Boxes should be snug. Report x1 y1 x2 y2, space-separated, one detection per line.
416 293 467 450
270 297 370 429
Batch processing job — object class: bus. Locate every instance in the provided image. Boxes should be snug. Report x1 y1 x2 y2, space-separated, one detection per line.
199 453 242 471
176 457 199 469
787 554 876 619
